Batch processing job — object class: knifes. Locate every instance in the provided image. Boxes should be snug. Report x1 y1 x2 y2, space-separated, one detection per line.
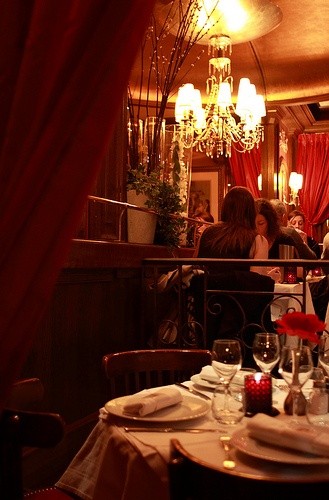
175 382 212 400
124 426 217 433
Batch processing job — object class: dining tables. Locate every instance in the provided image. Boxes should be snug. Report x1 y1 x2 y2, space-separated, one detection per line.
270 275 329 323
54 362 329 500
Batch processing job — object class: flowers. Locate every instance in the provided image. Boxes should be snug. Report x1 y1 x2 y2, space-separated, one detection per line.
112 0 222 252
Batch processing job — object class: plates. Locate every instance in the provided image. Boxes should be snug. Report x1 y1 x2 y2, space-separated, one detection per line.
272 378 315 413
104 396 211 422
190 371 255 390
231 419 329 463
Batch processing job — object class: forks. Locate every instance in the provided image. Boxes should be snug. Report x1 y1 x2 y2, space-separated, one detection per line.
218 430 235 469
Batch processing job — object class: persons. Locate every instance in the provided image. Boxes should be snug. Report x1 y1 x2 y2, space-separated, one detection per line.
255 198 329 259
192 185 282 285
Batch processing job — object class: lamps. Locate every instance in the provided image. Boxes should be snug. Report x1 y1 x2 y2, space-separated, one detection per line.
155 0 283 161
257 131 303 209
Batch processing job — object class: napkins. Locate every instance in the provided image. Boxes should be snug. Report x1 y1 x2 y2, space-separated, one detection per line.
122 384 183 417
246 412 328 456
200 365 252 386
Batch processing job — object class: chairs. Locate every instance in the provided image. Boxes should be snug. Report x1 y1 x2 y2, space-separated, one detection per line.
1 347 213 485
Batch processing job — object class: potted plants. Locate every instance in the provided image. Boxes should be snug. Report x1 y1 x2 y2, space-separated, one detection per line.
126 165 191 244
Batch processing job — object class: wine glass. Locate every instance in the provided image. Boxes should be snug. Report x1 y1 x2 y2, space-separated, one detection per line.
212 339 242 415
252 333 280 377
279 346 314 425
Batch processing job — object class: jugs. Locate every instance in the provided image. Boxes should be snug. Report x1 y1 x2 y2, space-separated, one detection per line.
278 243 299 283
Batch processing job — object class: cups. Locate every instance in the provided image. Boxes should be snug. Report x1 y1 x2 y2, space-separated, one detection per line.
211 386 246 424
235 367 256 386
306 392 329 429
298 365 326 400
318 336 329 378
